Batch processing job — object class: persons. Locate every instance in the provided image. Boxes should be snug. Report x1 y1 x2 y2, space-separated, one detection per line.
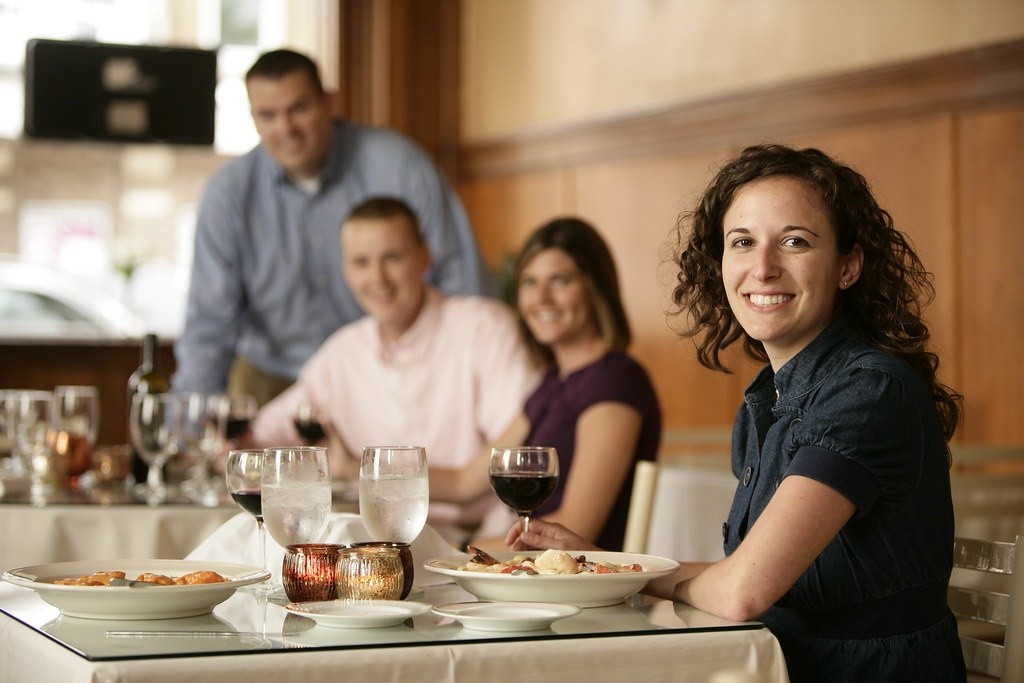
504 144 970 683
161 49 484 453
216 195 547 551
325 216 664 564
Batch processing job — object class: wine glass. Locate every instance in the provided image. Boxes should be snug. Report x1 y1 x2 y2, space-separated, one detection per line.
491 446 560 550
224 450 282 590
0 385 255 503
261 447 331 601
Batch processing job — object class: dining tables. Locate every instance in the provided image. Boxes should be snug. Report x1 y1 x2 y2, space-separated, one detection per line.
0 483 467 573
0 556 790 683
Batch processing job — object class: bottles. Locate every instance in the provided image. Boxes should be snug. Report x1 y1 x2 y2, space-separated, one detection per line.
125 333 170 483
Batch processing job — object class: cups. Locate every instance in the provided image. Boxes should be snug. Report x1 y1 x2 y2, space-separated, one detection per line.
360 446 430 543
294 397 327 448
283 542 415 601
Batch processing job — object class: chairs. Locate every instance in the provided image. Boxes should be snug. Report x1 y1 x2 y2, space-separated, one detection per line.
619 444 1024 683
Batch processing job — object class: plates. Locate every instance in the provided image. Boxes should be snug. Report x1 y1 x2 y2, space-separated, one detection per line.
39 614 272 656
3 557 270 617
423 548 681 609
430 599 583 631
282 599 432 626
